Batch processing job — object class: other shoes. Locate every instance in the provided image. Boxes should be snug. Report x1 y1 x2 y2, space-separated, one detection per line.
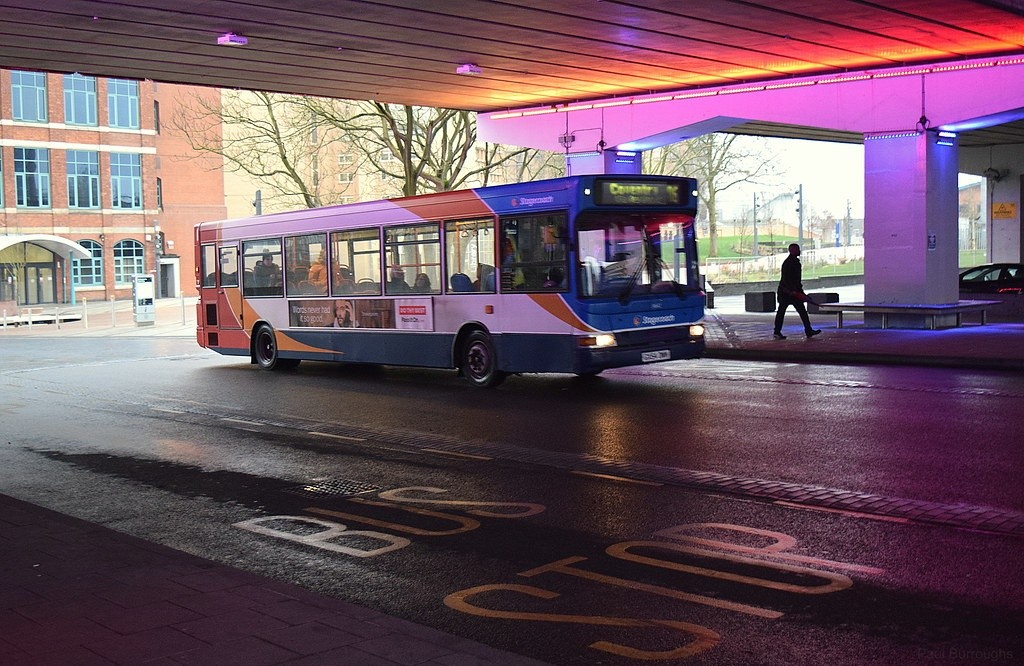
806 329 821 338
774 331 787 340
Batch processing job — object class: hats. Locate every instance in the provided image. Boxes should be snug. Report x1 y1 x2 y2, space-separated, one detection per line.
263 249 273 260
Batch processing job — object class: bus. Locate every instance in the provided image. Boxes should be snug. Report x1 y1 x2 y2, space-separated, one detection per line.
193 171 708 389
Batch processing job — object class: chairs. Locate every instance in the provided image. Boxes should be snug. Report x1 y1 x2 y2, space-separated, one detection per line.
339 264 351 279
294 266 309 282
338 279 357 294
358 278 377 292
244 268 255 287
298 280 314 292
279 266 296 283
275 281 296 293
450 273 475 292
208 272 228 286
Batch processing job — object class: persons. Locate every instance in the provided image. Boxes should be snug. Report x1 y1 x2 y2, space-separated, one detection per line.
473 263 496 291
325 300 362 328
411 273 431 292
549 262 565 284
285 253 311 285
253 250 283 288
388 264 410 293
309 248 345 294
773 244 822 340
504 223 525 289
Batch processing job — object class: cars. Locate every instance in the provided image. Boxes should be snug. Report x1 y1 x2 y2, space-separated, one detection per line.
959 263 1024 295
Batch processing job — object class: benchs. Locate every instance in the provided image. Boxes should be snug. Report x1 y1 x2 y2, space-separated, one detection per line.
819 299 1005 330
